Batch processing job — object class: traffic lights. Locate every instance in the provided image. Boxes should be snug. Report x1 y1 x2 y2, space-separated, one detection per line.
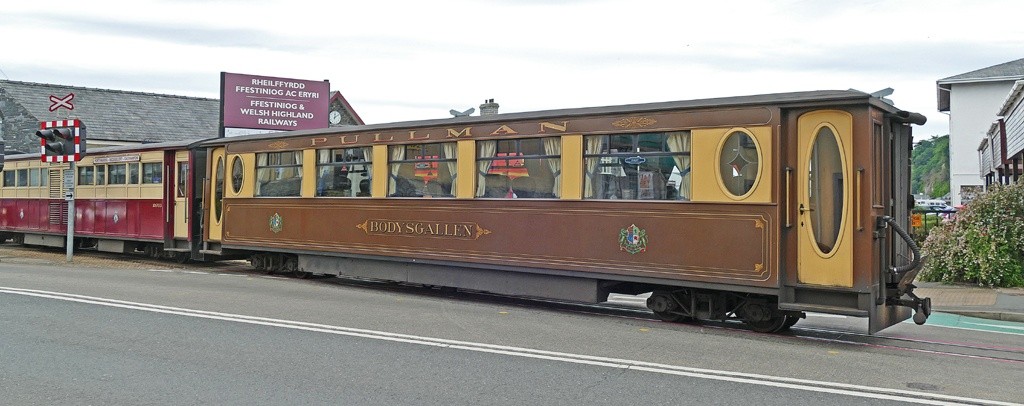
35 120 83 163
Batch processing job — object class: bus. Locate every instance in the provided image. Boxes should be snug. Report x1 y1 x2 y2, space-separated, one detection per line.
916 199 946 208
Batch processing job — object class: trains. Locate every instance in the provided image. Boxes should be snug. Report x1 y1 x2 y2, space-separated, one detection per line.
21 96 933 345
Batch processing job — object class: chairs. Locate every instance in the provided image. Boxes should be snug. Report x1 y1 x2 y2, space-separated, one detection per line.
334 173 679 200
